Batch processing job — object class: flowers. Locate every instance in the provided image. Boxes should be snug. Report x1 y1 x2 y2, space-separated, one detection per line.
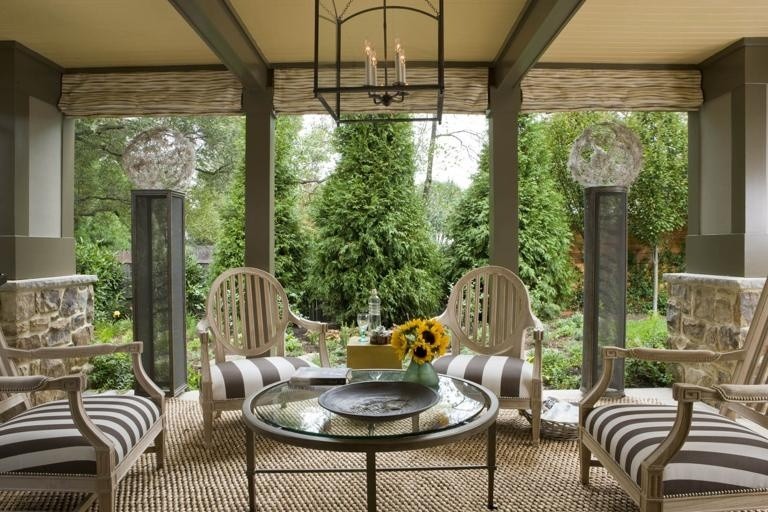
387 316 453 382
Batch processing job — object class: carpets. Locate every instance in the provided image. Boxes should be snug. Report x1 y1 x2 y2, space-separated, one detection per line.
0 397 768 511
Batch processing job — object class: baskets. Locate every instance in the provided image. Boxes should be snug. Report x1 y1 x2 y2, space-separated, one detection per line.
518 398 579 441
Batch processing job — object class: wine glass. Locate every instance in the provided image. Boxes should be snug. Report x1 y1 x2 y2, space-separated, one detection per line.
357 313 370 342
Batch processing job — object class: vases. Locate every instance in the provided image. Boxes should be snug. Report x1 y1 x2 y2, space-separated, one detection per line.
396 355 442 387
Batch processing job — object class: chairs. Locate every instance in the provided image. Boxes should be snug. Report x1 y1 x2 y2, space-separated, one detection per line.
578 280 767 511
197 266 331 447
430 262 545 447
0 326 166 512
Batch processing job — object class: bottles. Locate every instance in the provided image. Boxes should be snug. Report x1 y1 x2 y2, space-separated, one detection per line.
368 289 381 331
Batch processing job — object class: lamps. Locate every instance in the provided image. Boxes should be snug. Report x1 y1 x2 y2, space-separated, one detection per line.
314 0 447 126
